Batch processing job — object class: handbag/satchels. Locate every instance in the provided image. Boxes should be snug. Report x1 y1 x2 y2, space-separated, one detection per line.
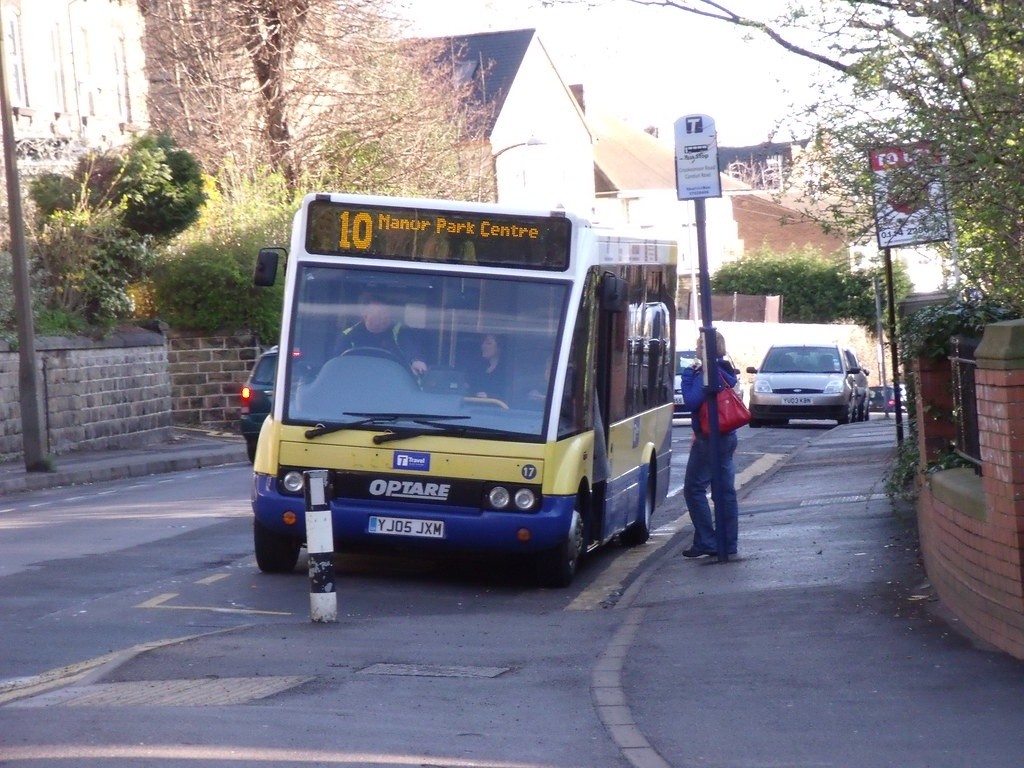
699 372 753 437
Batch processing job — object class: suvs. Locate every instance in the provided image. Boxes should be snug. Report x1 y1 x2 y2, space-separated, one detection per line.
674 346 745 411
239 344 317 465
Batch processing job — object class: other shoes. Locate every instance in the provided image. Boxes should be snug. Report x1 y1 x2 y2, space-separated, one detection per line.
708 551 737 556
682 548 717 557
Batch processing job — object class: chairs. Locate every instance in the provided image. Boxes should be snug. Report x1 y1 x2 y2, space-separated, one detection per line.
779 355 795 369
818 355 835 371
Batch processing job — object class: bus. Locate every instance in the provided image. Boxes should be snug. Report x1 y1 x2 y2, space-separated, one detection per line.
250 191 678 587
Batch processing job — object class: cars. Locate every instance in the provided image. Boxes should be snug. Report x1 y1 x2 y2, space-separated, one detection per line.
746 347 862 424
866 385 908 413
846 349 872 420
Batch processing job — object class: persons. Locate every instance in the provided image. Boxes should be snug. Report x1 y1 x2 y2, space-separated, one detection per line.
679 329 739 556
333 289 430 389
464 336 530 408
